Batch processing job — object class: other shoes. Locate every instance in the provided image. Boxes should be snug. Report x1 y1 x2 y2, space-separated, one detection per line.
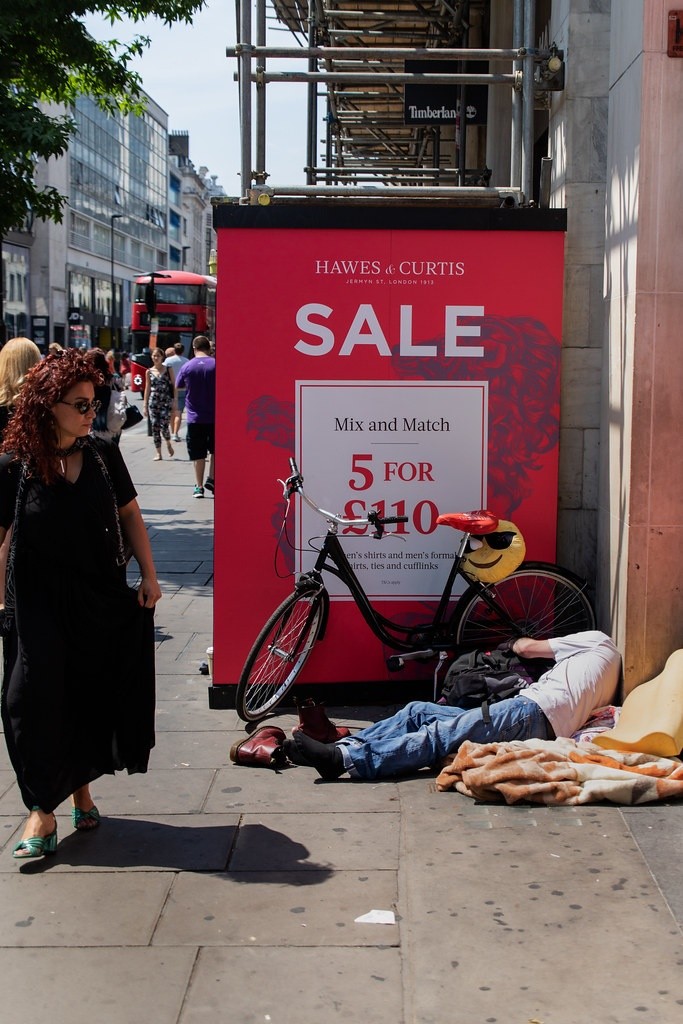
167 446 174 457
171 434 179 442
152 455 162 461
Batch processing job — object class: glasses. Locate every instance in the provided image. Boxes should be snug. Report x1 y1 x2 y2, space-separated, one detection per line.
57 399 102 414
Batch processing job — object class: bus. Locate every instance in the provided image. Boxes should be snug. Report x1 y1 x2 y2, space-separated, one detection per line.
127 269 217 399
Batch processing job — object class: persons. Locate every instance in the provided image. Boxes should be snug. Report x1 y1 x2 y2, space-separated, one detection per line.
0 337 44 446
282 630 622 781
40 343 64 359
0 348 163 858
79 343 191 461
175 336 215 498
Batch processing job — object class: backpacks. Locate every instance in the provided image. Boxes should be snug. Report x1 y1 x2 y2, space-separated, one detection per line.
436 646 548 723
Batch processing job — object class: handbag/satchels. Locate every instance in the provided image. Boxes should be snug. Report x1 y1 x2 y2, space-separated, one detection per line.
105 385 125 433
120 401 143 430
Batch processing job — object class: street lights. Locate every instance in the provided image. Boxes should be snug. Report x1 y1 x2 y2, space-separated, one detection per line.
111 214 123 349
181 246 191 271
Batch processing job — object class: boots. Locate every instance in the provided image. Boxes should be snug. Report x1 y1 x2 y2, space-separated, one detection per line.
290 696 351 744
230 725 287 769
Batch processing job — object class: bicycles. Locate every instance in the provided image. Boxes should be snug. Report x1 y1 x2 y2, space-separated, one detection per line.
236 455 600 724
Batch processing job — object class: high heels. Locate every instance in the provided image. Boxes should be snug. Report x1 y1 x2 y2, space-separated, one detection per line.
12 805 58 858
71 804 101 832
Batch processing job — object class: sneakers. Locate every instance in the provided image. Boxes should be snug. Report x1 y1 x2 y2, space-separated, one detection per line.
204 476 214 494
191 485 204 497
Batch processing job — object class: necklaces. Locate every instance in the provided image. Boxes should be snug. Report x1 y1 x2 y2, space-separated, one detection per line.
53 437 83 474
